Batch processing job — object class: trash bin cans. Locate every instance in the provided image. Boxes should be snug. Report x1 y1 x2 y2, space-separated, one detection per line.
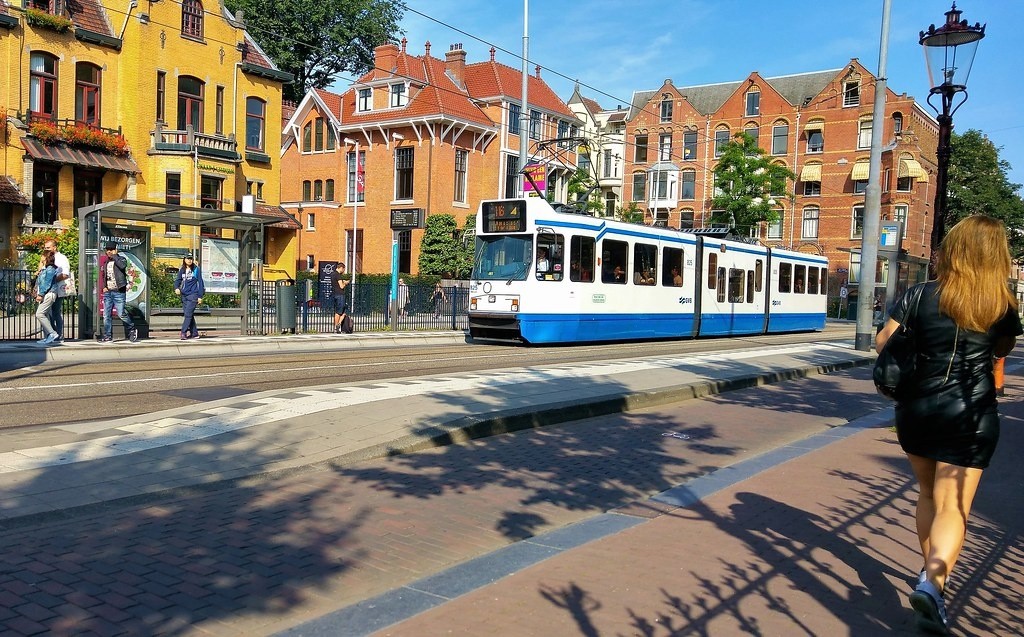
275 278 296 328
846 290 858 320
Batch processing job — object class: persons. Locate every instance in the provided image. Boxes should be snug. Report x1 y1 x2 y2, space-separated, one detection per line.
331 263 351 334
874 214 1020 634
36 240 71 343
532 247 817 296
174 251 205 340
34 250 61 345
387 278 410 319
97 241 138 343
430 282 448 318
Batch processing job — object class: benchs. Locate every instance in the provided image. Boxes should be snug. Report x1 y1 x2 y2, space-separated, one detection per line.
150 304 211 316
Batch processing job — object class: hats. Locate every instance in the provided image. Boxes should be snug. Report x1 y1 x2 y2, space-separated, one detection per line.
184 252 193 258
104 241 116 249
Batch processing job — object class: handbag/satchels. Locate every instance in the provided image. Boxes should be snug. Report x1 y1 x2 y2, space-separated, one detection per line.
873 283 926 400
341 316 353 333
57 273 77 298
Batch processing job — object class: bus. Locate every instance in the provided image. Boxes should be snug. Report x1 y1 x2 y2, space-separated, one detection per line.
462 137 830 345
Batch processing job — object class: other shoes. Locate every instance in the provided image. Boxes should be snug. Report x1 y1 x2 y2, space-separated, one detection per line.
102 337 112 342
336 324 342 334
334 329 337 333
188 332 199 339
52 338 64 343
131 328 138 342
181 334 187 339
37 339 47 344
46 333 59 343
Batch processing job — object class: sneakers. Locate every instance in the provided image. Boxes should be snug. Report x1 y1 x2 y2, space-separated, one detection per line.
918 567 950 583
908 581 948 637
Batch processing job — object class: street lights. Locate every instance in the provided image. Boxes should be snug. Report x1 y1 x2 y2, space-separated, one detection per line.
919 1 988 281
